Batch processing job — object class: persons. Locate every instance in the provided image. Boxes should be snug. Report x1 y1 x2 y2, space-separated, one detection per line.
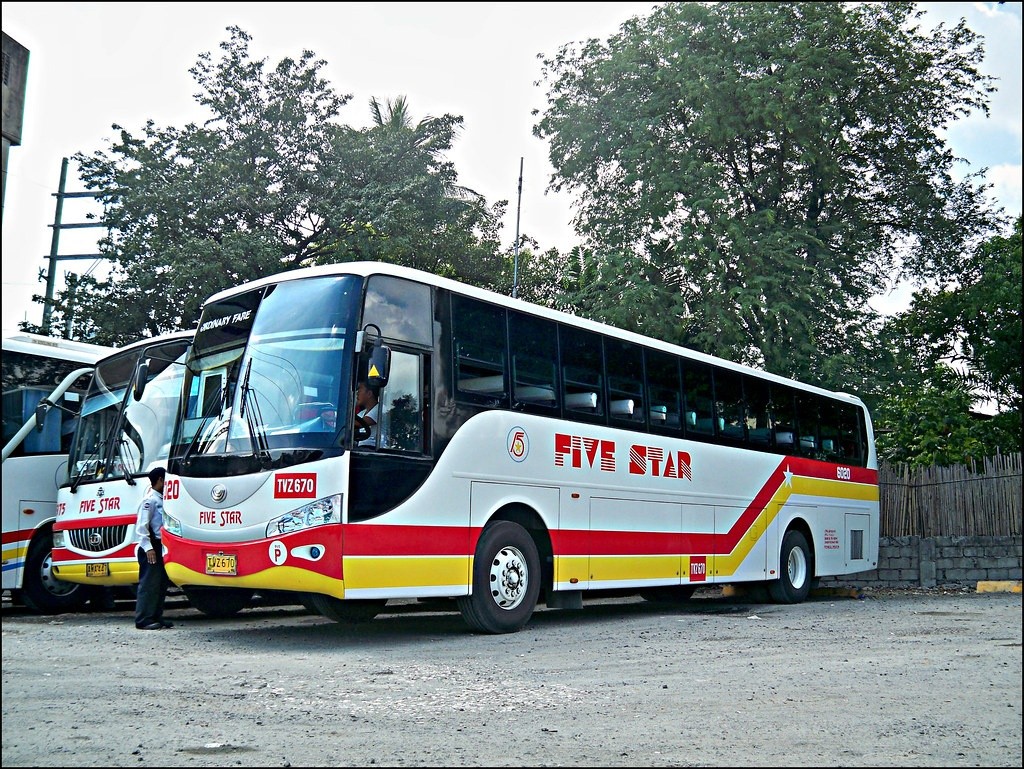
135 467 174 631
320 379 387 449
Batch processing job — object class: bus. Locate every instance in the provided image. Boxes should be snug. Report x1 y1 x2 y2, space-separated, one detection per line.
0 331 122 615
36 328 254 617
131 259 882 632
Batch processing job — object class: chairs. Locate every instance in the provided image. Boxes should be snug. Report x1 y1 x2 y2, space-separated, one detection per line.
459 374 835 452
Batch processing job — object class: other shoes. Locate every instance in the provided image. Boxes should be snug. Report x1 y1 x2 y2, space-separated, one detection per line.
136 620 172 629
